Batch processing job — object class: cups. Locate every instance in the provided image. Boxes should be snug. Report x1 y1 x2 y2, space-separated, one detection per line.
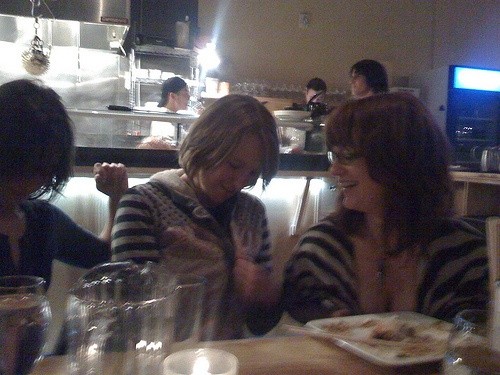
170 273 206 353
67 266 179 375
163 347 237 375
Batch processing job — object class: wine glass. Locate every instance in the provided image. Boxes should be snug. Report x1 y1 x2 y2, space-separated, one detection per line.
0 275 51 375
443 309 499 375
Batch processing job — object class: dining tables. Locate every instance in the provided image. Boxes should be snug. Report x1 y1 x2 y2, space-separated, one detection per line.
30 335 500 375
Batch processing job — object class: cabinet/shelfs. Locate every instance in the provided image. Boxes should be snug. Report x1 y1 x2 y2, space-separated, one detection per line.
130 47 199 136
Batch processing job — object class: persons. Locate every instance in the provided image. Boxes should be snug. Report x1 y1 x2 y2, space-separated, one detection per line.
111 94 284 341
150 78 206 147
283 92 488 325
0 79 129 297
349 59 389 99
284 78 327 110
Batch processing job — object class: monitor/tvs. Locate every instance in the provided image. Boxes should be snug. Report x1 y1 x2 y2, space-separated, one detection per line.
445 65 500 161
116 0 198 57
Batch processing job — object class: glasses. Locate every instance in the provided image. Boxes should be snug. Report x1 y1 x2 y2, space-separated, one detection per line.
326 150 364 165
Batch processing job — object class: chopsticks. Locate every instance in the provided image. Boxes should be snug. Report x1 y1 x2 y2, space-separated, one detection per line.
282 324 427 347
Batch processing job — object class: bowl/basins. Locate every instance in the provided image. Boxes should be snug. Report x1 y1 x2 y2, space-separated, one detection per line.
274 110 311 122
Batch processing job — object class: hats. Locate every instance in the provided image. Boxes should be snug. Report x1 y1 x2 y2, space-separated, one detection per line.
157 77 186 107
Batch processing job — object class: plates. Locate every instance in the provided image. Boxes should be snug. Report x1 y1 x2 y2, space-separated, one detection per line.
308 312 483 367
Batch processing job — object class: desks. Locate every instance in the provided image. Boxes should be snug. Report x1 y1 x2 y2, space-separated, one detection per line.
450 171 500 216
